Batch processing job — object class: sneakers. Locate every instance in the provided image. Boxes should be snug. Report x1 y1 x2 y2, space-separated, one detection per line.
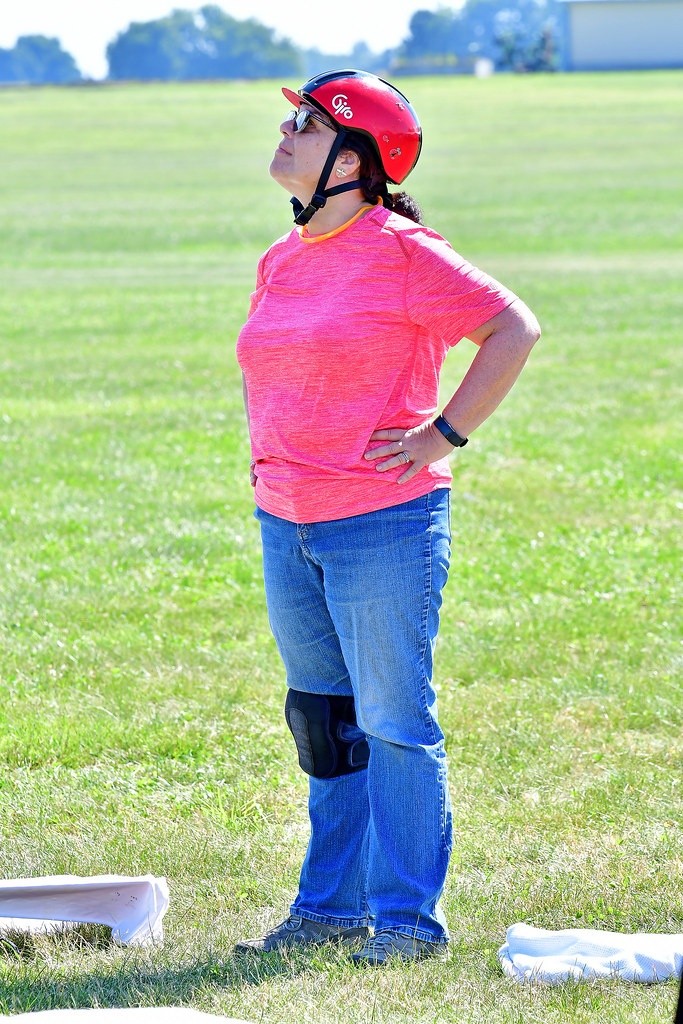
234 909 372 959
347 932 453 969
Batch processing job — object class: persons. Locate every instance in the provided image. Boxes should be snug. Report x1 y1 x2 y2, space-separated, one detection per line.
235 69 542 966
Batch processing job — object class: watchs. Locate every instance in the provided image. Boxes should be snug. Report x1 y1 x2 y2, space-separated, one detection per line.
433 415 469 447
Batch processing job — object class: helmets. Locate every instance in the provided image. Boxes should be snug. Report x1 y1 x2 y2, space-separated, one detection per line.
282 68 422 184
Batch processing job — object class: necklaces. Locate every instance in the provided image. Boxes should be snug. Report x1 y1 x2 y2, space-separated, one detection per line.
299 196 383 244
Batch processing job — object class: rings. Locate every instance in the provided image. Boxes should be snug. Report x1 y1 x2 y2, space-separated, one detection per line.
403 452 410 463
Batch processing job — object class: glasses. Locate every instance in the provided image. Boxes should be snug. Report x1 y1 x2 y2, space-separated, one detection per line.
285 111 338 133
